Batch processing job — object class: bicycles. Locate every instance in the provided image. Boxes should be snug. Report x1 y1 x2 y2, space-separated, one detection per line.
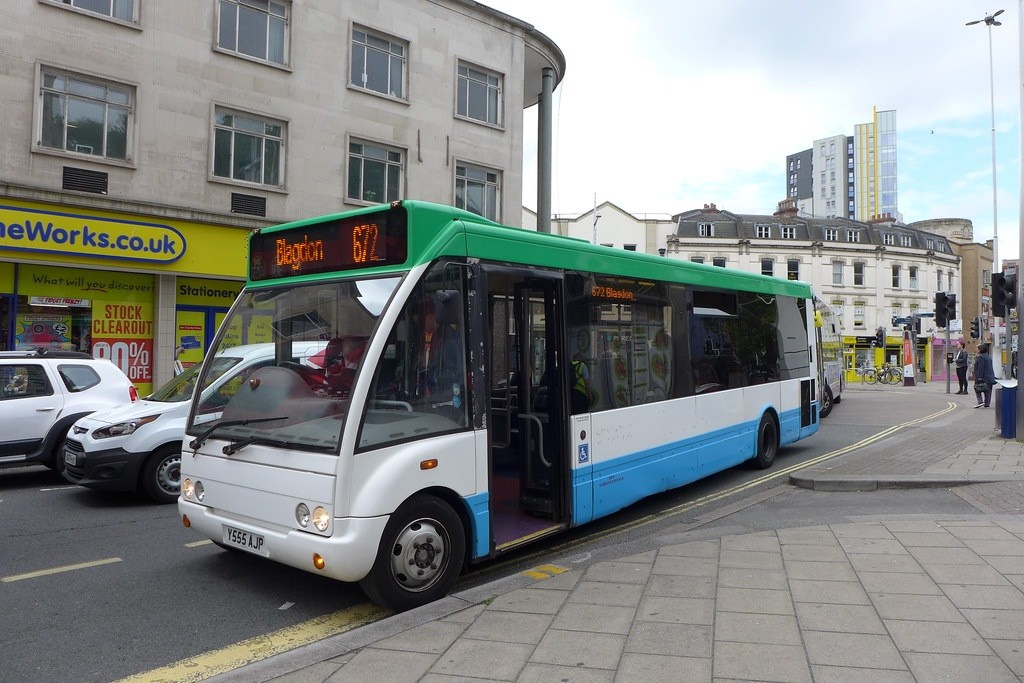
863 362 902 385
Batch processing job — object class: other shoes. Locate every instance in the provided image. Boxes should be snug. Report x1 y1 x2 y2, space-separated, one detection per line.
985 405 989 407
960 391 968 395
955 391 961 394
975 403 984 408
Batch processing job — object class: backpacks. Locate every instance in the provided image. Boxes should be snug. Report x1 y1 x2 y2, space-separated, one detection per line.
570 360 583 385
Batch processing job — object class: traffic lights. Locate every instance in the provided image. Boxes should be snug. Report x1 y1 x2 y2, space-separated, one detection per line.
946 352 950 366
999 274 1016 309
877 328 884 348
971 317 982 339
942 293 957 320
903 323 913 331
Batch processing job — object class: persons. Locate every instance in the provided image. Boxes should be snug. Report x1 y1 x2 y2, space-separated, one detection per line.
3 375 28 391
571 352 590 412
955 342 968 395
974 344 998 408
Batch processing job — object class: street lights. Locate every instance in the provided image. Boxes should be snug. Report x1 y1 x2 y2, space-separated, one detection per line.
965 10 1007 370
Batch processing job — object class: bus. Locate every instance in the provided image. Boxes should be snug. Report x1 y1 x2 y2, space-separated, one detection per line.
177 199 822 620
812 294 844 418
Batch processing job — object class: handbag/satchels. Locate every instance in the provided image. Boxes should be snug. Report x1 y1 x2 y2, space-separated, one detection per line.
975 379 988 392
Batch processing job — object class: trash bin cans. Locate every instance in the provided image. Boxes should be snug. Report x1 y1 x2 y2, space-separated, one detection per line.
995 388 1003 430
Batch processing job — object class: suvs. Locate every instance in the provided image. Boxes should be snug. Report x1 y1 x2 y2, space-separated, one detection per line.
0 349 139 476
57 340 398 507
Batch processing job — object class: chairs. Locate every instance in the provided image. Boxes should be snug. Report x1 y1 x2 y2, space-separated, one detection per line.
535 366 558 412
497 370 534 415
